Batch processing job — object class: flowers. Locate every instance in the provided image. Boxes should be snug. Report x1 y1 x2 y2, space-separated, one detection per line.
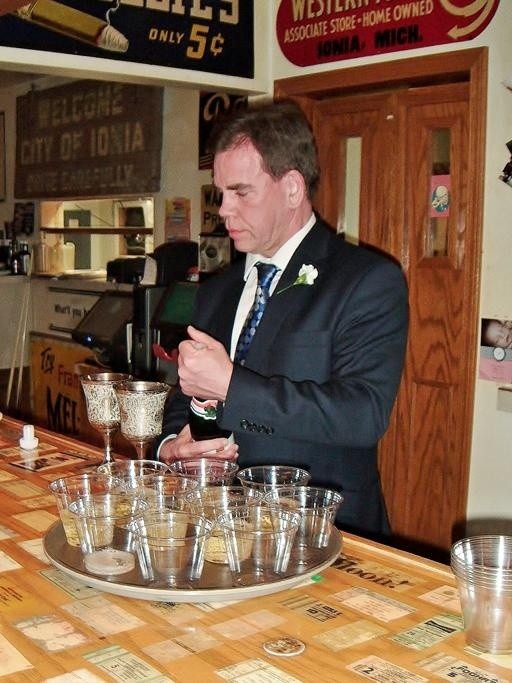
276 261 319 296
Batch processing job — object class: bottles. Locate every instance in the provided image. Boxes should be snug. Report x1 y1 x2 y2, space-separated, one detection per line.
7 238 32 276
34 232 65 276
189 343 238 454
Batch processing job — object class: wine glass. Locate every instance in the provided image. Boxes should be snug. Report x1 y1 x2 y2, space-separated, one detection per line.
112 378 171 474
77 372 134 475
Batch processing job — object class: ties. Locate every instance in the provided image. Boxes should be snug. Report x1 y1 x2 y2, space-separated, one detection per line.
232 260 283 370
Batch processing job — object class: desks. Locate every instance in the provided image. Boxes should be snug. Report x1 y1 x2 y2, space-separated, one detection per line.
0 413 512 683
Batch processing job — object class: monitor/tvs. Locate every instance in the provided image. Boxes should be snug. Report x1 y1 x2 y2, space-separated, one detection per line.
70 289 135 348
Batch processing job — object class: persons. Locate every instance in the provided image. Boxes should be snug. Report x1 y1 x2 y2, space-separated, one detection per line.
151 96 410 549
481 316 511 350
432 184 449 211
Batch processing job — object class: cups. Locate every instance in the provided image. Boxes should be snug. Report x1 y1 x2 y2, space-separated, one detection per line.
68 492 151 576
47 456 344 566
125 513 214 590
217 508 303 587
451 534 512 655
